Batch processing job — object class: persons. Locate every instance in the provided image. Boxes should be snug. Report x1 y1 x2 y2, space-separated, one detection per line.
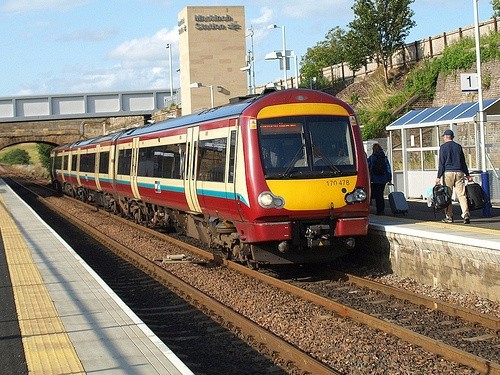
312 144 332 166
367 144 391 215
260 142 284 168
435 129 473 224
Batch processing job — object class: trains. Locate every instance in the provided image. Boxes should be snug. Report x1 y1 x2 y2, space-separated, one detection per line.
48 89 371 273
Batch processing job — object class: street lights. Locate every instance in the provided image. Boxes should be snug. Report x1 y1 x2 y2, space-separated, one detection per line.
268 24 288 88
165 42 173 102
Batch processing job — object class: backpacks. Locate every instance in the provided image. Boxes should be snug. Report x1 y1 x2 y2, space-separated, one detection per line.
372 151 386 175
432 183 450 210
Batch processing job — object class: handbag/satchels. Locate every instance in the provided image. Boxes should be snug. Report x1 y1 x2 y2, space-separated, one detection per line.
465 180 486 211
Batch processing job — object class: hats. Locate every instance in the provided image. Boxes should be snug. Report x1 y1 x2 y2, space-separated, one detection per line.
441 130 453 137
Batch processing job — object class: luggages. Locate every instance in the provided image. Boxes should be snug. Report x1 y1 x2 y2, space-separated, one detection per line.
387 184 409 215
